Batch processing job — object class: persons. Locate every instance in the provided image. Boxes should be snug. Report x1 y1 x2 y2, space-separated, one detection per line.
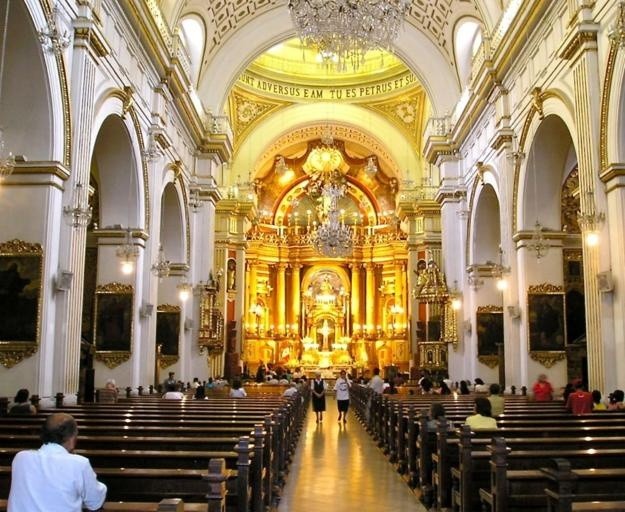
305 369 355 425
478 317 497 353
358 367 625 432
6 412 108 511
153 359 310 411
319 276 333 295
8 388 37 415
533 303 563 348
228 261 236 290
106 379 116 390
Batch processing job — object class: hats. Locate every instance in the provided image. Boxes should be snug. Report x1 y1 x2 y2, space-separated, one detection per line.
572 377 583 386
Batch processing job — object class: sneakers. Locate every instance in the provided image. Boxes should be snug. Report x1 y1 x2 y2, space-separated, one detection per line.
338 413 347 423
316 415 323 423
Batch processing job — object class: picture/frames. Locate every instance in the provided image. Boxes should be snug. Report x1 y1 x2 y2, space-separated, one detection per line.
0 239 180 368
526 282 565 368
476 305 503 369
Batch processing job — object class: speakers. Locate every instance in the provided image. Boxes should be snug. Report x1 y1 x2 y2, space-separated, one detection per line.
596 271 615 292
184 318 194 329
55 270 73 291
144 305 153 316
507 306 516 317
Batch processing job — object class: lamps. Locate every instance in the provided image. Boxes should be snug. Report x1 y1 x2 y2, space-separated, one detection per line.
116 157 140 275
287 0 411 73
526 144 551 263
309 169 353 257
491 245 515 289
575 191 605 246
152 195 171 280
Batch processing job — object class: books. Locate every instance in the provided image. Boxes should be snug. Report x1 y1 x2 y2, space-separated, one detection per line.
158 498 183 512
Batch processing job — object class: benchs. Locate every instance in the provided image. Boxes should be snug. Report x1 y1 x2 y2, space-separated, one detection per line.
350 385 625 512
